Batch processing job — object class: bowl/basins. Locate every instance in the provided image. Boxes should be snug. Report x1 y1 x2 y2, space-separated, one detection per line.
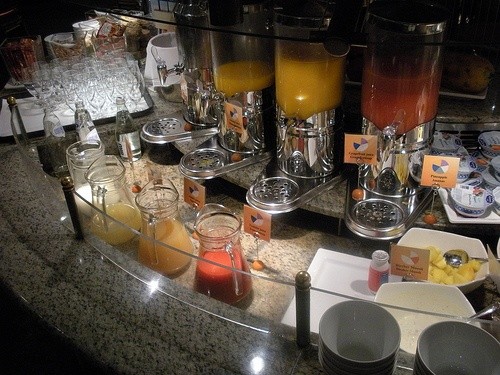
413 320 500 375
372 282 482 358
317 300 402 375
430 124 500 217
392 227 490 296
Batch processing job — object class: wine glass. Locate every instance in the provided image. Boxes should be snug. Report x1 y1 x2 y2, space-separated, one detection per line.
20 43 147 124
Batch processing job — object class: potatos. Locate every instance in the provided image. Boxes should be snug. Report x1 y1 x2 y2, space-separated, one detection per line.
424 246 482 285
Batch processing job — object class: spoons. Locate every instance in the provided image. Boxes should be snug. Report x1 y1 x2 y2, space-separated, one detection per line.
443 249 500 269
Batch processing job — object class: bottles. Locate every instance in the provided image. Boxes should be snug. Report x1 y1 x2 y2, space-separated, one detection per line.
146 32 185 102
368 248 390 293
112 96 143 163
42 107 66 138
73 100 100 147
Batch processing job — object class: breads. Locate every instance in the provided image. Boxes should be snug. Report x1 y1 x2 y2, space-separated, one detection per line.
440 55 495 94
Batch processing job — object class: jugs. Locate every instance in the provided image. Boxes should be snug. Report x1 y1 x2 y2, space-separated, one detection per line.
42 139 254 311
